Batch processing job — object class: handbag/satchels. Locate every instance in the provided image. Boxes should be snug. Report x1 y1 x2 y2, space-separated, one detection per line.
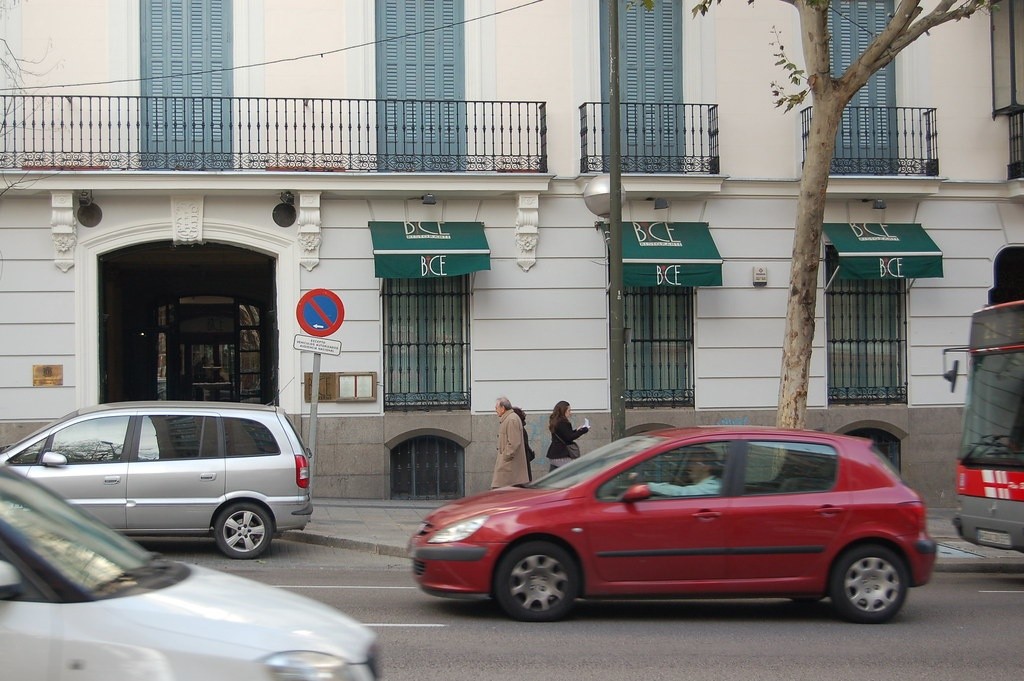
528 447 535 461
567 442 580 458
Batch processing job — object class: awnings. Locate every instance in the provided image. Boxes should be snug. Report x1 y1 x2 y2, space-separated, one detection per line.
822 223 944 294
368 221 491 296
594 220 724 293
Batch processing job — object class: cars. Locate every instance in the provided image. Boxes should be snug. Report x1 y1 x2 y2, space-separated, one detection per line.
0 461 384 681
405 420 940 627
0 400 317 561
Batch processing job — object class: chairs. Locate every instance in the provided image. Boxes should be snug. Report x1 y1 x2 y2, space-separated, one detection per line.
776 475 804 493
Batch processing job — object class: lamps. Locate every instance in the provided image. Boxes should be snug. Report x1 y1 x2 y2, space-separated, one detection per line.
272 191 297 227
646 197 668 209
421 193 436 204
862 198 887 209
77 190 102 227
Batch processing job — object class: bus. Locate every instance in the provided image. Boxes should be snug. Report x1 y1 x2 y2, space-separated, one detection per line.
941 295 1024 556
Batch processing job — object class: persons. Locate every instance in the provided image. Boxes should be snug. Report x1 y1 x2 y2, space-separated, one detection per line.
546 401 591 472
491 397 529 489
628 447 723 495
512 406 532 482
